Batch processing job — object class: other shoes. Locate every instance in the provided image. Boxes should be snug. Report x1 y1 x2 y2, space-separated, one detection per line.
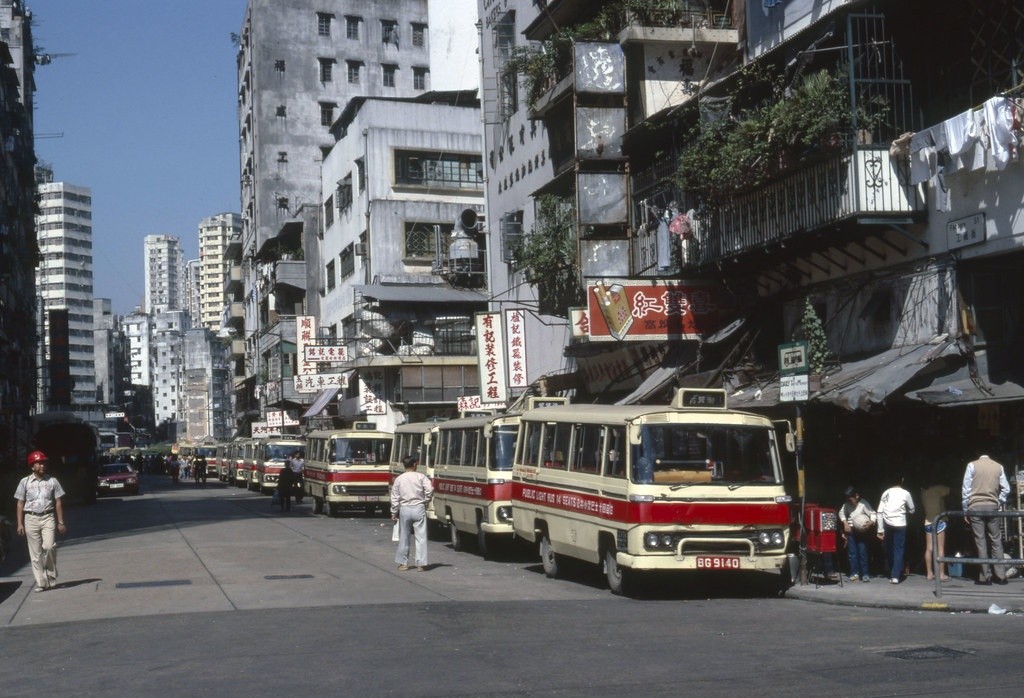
999 579 1009 584
417 567 423 572
48 575 56 587
398 564 408 570
850 574 859 581
861 574 869 582
975 578 992 585
35 587 46 591
890 577 899 583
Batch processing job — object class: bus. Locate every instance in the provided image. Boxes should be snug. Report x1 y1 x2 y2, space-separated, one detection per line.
511 387 796 595
109 438 306 494
301 421 396 516
389 397 568 559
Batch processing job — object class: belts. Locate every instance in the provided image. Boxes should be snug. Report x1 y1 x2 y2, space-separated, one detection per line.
25 510 54 515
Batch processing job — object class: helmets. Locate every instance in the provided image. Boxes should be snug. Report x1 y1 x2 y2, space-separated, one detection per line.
28 451 49 464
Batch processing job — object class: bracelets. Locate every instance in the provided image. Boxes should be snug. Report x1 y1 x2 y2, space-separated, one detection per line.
58 521 64 525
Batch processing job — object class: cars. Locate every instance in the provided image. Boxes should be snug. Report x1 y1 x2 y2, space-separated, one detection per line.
96 464 139 497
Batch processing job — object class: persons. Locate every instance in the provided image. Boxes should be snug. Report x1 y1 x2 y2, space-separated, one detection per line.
13 451 66 592
390 456 434 571
837 447 1020 587
277 451 304 512
98 451 207 484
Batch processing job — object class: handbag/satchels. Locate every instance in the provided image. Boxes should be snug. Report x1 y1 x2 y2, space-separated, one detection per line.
273 491 280 505
392 519 399 542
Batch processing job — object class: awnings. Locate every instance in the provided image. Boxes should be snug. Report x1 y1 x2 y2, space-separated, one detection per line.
300 368 357 417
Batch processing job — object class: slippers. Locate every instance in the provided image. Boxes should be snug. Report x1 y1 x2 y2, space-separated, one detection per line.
926 574 935 581
941 575 952 582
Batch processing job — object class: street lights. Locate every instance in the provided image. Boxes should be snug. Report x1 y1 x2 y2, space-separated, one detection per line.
190 375 225 440
228 329 284 439
184 388 211 436
